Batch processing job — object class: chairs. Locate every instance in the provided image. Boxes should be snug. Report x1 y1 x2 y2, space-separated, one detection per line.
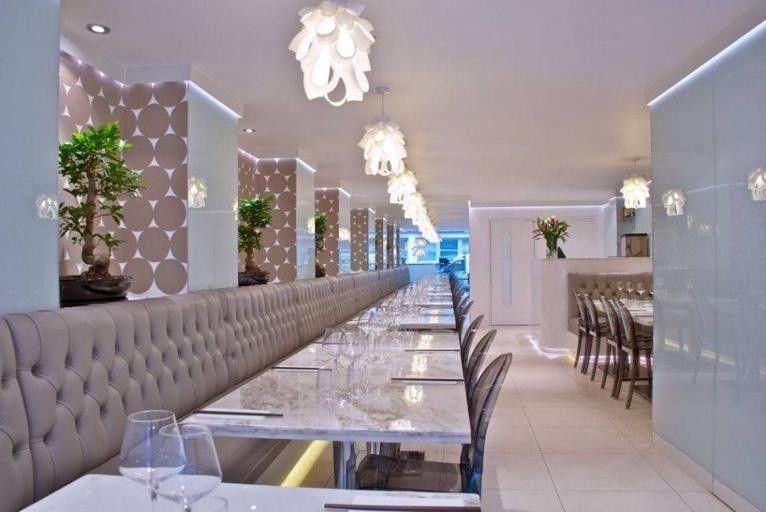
352 271 510 493
569 286 655 409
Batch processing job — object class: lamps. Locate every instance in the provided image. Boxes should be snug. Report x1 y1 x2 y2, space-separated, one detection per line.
619 158 653 209
288 0 375 107
356 88 443 243
661 189 685 216
747 168 766 201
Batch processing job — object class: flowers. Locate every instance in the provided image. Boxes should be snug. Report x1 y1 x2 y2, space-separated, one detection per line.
529 214 571 259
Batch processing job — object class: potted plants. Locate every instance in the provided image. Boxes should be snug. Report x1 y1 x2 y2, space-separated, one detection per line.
314 212 327 277
59 120 146 307
238 194 274 285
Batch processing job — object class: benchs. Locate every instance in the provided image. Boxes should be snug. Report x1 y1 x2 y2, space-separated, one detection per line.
0 266 409 512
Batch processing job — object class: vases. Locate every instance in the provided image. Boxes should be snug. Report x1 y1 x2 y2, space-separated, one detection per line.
546 239 558 261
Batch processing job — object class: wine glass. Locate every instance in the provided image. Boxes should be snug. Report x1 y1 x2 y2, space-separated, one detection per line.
616 280 646 309
119 409 224 512
321 281 432 371
